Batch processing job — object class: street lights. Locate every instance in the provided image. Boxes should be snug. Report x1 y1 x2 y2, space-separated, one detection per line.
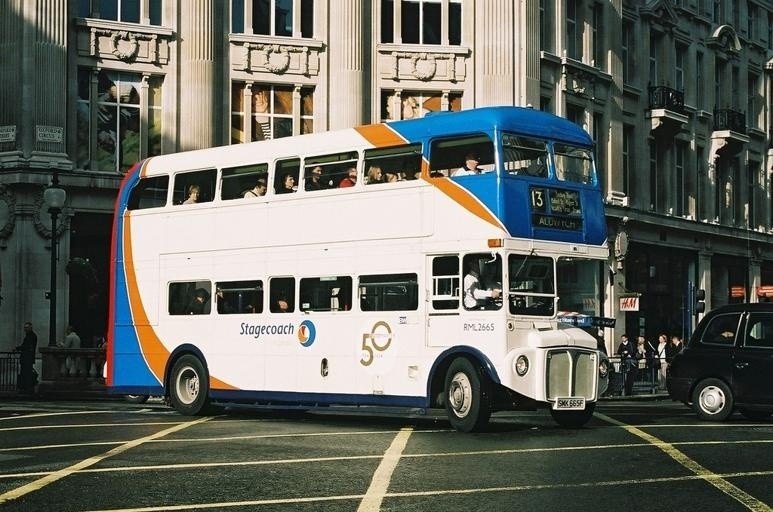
43 169 67 377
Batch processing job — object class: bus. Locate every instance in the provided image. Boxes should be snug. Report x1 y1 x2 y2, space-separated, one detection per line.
103 107 611 432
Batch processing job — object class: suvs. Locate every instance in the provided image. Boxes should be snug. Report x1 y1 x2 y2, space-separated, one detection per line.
666 302 772 422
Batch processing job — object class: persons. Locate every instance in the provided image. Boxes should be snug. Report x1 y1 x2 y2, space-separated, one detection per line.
183 286 291 314
243 82 297 141
384 92 429 122
242 176 267 198
448 149 485 176
180 184 201 205
670 335 683 353
705 318 735 345
381 171 399 182
303 161 333 191
634 334 652 382
365 164 386 186
526 157 546 177
273 173 297 194
653 335 671 391
58 324 81 376
338 164 357 187
10 320 37 394
615 334 635 396
462 263 501 311
401 161 419 181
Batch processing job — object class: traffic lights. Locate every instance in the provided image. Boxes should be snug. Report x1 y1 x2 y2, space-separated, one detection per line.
692 286 705 315
